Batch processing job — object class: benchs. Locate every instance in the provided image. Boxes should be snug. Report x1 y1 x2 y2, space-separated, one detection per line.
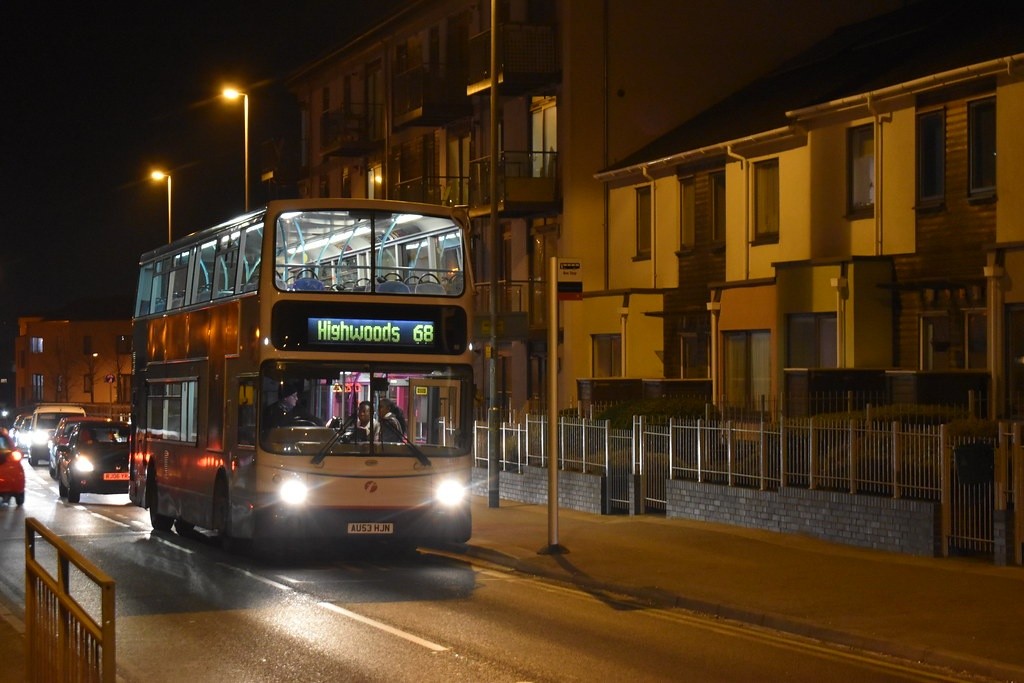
146 268 447 314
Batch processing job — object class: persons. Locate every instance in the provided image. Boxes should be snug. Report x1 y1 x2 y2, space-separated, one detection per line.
349 400 380 443
376 399 407 442
273 383 311 427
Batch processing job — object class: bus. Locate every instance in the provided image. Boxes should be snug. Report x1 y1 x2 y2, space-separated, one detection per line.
128 199 476 555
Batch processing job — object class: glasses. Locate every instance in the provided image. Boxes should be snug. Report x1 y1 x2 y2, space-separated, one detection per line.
291 394 298 398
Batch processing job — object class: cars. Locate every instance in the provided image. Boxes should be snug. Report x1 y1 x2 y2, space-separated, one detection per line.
56 421 129 503
0 429 25 504
48 416 113 479
9 405 88 467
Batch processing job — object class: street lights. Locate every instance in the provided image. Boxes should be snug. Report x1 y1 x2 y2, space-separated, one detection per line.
222 89 253 214
149 170 174 245
88 352 99 402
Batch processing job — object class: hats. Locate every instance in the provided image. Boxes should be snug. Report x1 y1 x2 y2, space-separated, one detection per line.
280 381 299 398
